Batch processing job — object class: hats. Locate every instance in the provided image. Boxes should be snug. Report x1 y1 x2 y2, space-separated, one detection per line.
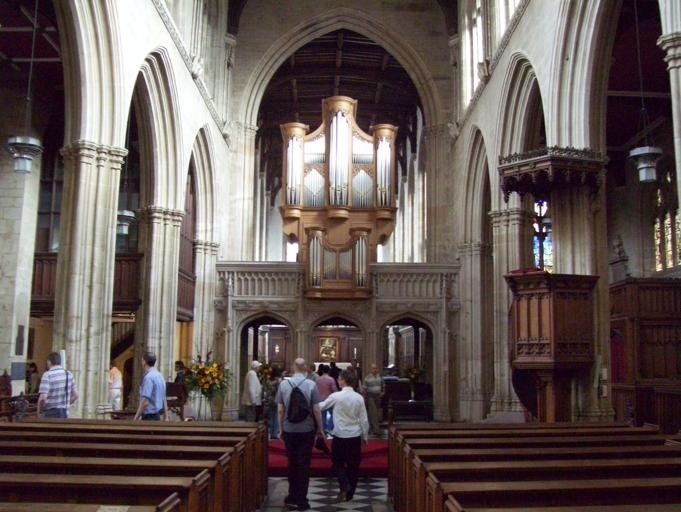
252 361 262 368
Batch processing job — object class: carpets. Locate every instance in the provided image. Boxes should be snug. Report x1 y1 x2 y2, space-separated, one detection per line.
264 432 390 477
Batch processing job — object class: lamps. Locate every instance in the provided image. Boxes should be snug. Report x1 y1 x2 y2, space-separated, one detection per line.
626 1 665 187
7 0 46 176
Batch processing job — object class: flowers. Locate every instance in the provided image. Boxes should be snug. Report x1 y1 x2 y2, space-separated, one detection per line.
178 336 232 401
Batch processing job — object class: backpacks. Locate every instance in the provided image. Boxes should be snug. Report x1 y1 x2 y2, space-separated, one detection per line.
286 379 310 423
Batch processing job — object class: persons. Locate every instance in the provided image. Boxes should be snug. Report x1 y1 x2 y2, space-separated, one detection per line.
132 350 172 422
316 368 371 502
110 360 127 420
275 356 326 511
175 360 188 386
35 352 80 420
25 361 43 395
234 360 402 440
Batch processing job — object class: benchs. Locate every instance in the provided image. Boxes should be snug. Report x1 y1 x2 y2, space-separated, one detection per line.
381 416 675 508
1 408 274 510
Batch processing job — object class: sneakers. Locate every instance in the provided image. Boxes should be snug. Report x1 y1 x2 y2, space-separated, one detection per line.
338 486 353 501
285 494 309 511
